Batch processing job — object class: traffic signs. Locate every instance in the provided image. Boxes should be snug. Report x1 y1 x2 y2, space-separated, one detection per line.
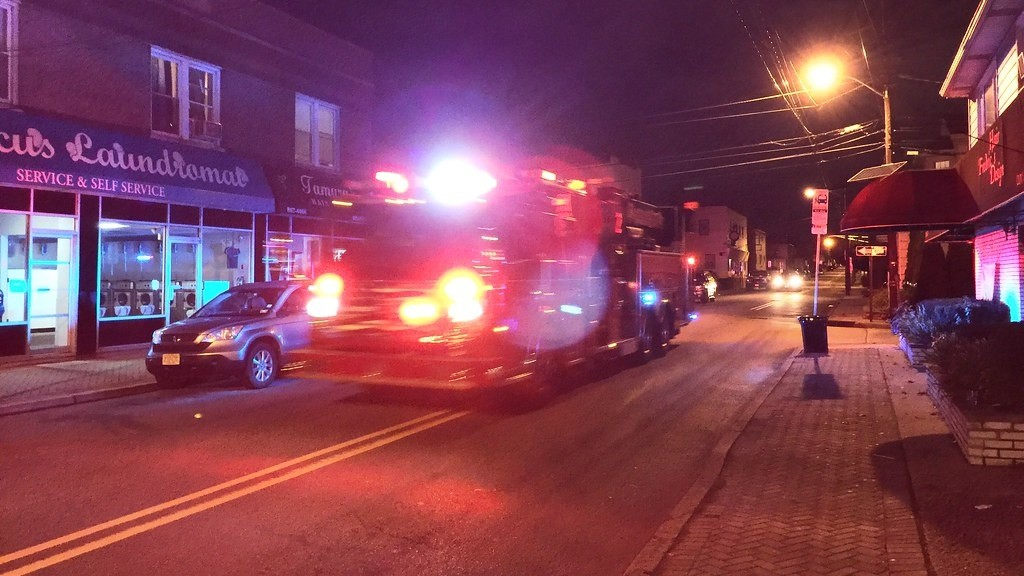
856 244 887 257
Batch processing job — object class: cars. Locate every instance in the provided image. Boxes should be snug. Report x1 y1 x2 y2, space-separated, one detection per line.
690 271 718 304
747 265 804 291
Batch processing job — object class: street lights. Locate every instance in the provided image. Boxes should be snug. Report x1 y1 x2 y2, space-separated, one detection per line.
805 59 900 323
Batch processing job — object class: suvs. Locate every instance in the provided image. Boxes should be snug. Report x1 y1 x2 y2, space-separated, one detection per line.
146 281 315 390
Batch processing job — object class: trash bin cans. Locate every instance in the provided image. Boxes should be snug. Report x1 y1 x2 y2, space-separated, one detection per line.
797 315 830 353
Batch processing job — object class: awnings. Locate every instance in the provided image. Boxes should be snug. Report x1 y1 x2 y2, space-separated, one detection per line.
257 154 372 224
840 168 965 232
0 109 276 214
729 248 749 261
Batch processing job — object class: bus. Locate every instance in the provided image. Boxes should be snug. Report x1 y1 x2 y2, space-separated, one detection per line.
308 168 696 398
